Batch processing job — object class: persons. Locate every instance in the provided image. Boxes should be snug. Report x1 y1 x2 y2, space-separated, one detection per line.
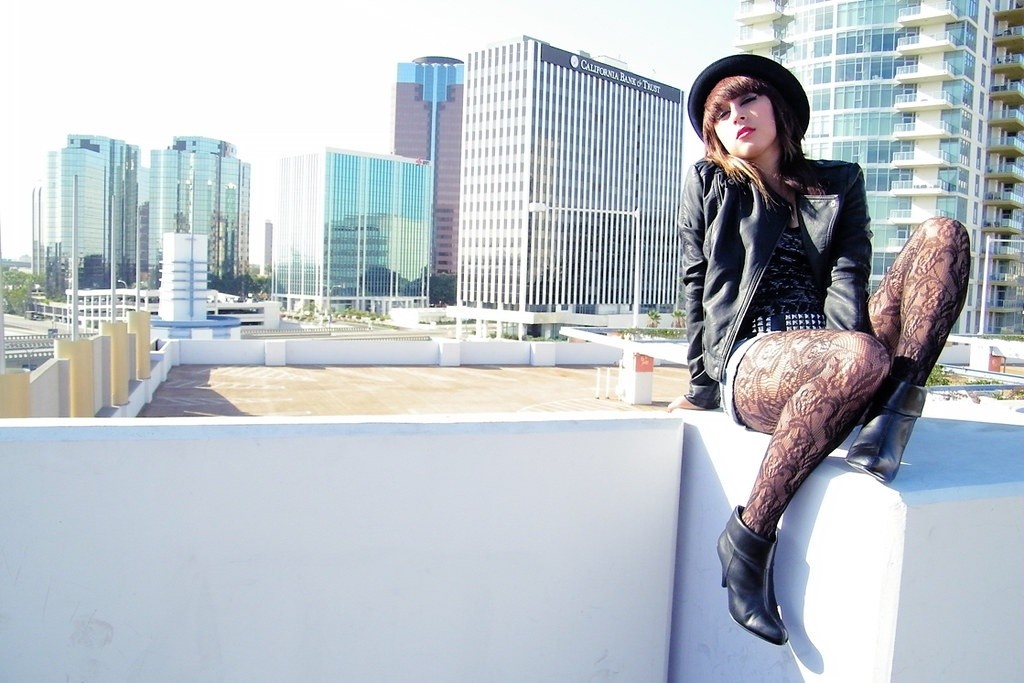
666 53 971 646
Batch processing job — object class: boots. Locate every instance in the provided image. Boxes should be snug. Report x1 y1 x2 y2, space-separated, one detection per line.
715 505 789 646
843 376 928 482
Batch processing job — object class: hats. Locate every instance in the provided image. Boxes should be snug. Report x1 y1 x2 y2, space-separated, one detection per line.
687 53 812 141
